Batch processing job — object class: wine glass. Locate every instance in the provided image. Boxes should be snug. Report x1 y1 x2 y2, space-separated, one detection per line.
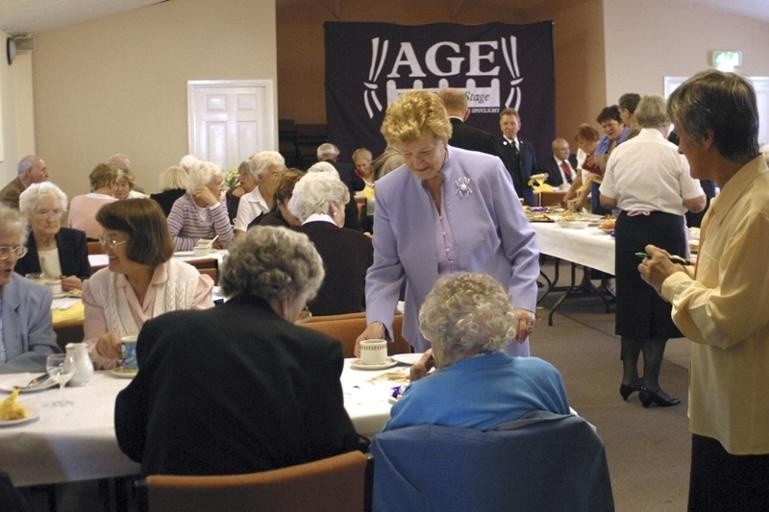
45 352 78 408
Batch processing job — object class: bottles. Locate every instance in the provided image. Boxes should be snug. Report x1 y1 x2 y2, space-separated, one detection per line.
61 342 95 388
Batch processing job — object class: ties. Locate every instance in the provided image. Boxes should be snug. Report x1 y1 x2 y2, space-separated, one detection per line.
511 141 519 154
561 161 574 184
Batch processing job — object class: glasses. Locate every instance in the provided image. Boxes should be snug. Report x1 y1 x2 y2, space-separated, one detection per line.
0 246 28 261
99 234 128 249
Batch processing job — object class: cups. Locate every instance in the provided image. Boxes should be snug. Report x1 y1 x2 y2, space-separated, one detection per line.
112 335 141 371
192 237 213 259
359 338 389 366
44 279 62 296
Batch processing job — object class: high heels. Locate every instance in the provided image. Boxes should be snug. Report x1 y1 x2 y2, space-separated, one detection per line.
639 388 680 408
620 379 641 401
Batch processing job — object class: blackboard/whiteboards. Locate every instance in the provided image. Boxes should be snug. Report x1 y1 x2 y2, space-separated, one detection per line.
663 78 769 147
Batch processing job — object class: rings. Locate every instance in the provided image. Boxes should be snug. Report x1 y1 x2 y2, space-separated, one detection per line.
525 324 534 334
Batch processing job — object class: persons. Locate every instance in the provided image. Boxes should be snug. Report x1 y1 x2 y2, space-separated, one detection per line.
1 140 377 372
596 96 707 408
496 109 549 204
382 271 568 431
544 93 717 231
437 86 524 198
115 226 373 511
350 90 541 357
372 142 406 182
634 68 768 512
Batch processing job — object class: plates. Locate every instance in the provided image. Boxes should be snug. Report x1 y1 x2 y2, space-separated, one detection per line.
68 291 82 298
48 292 67 299
522 204 615 235
390 351 426 365
107 367 139 378
350 355 397 370
0 406 39 428
0 373 59 394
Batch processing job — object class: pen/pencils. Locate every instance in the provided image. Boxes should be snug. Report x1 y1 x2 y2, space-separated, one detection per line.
633 250 695 266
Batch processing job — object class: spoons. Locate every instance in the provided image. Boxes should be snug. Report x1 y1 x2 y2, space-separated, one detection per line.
12 373 49 392
